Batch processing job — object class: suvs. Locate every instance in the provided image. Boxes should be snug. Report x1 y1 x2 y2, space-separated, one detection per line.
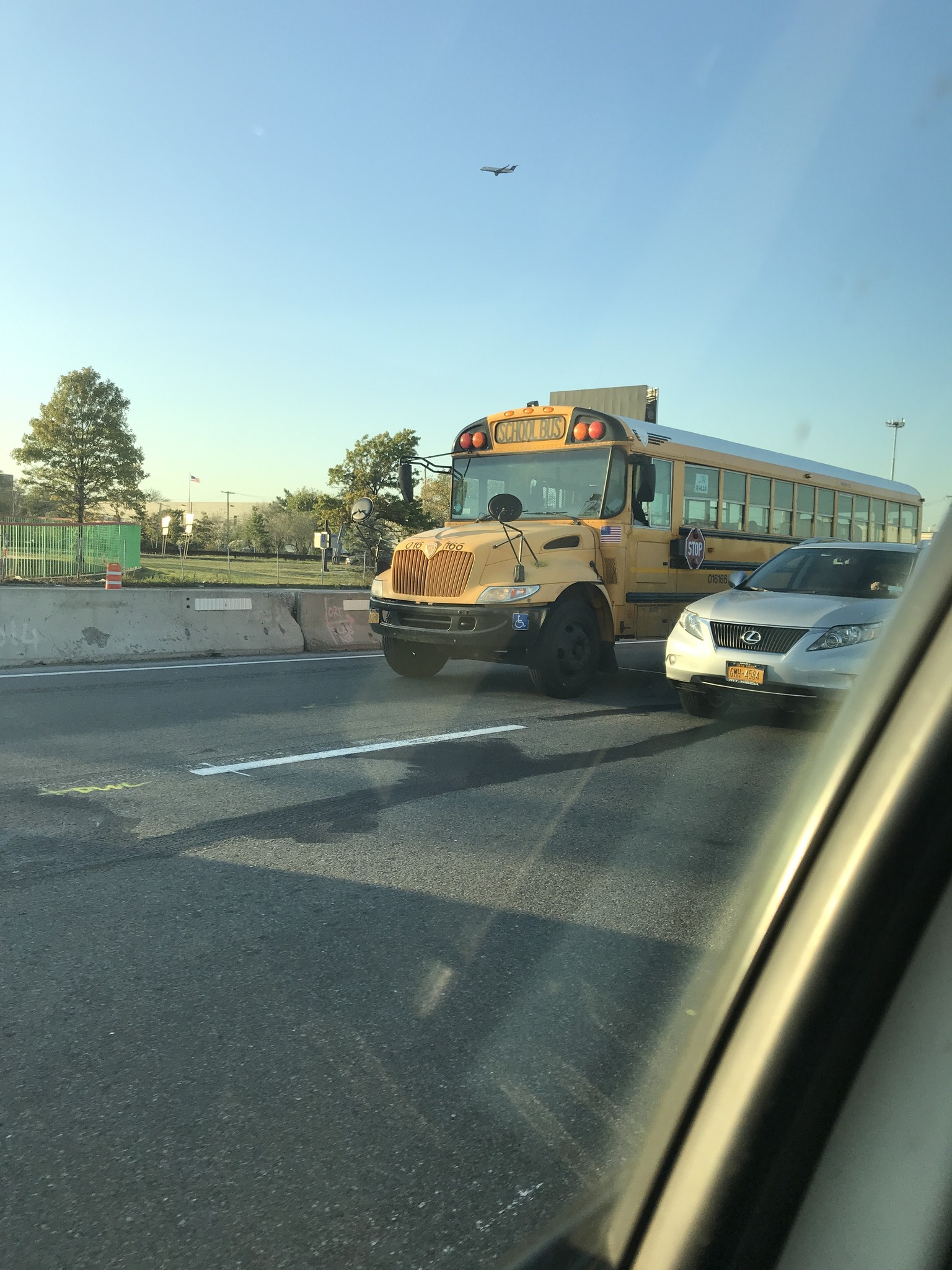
664 537 932 716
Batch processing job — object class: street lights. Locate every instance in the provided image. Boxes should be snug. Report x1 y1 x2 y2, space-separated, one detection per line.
885 417 906 481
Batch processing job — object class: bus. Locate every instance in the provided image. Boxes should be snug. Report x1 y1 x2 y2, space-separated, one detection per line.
350 400 924 702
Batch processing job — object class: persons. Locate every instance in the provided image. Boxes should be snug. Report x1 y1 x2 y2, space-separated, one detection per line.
581 464 647 526
870 561 905 593
